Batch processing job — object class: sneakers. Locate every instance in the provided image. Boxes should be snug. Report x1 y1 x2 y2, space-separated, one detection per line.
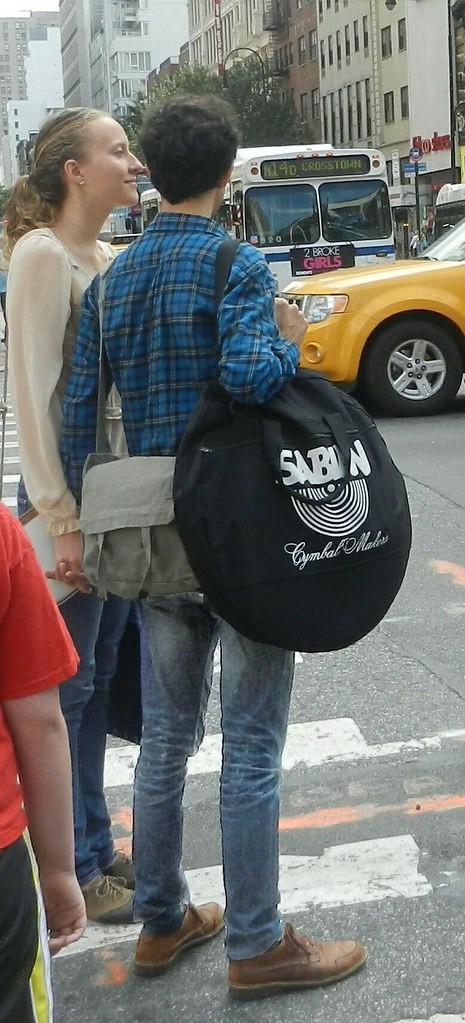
100 847 136 889
228 922 368 999
82 873 136 924
133 901 226 977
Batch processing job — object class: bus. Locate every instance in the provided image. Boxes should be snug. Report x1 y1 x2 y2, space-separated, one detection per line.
97 143 399 292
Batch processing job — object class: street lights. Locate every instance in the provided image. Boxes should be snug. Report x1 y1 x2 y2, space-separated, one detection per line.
221 46 270 145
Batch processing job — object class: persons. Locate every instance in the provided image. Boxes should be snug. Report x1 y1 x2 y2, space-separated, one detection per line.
0 508 86 1023
7 107 143 926
409 227 428 257
44 95 367 999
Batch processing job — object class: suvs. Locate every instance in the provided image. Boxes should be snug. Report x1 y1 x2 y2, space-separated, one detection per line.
276 216 465 419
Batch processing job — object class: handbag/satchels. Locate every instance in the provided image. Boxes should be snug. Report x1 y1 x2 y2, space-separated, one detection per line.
174 235 408 653
19 505 79 607
79 450 205 603
